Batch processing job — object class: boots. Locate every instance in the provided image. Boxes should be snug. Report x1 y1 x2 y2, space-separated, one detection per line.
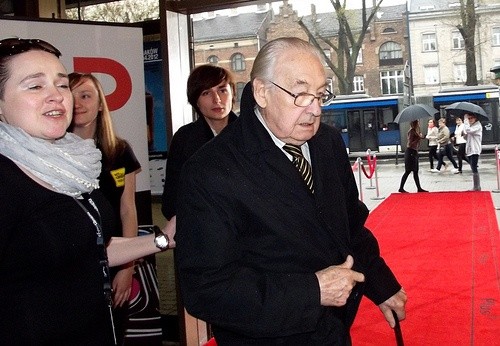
468 174 481 192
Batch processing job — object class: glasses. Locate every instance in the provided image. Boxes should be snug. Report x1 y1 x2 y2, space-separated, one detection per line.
268 79 336 108
0 38 62 57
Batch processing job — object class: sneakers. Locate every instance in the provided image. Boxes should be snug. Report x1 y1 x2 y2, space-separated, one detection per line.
444 164 449 170
425 168 440 173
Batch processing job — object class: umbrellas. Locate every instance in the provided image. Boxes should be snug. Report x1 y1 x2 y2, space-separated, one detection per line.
445 101 490 123
393 103 441 135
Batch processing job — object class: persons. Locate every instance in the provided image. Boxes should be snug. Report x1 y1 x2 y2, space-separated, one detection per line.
425 116 479 174
1 38 178 346
159 36 408 346
461 111 483 191
398 120 430 193
64 72 142 346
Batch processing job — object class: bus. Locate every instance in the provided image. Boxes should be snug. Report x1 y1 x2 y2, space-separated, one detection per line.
320 85 500 158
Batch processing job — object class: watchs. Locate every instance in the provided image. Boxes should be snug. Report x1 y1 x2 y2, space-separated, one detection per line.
152 226 171 252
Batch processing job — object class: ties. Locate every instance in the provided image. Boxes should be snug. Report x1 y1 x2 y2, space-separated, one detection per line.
282 143 314 197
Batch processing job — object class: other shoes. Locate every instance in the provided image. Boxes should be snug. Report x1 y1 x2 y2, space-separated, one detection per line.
451 168 463 174
398 189 408 193
417 190 428 192
